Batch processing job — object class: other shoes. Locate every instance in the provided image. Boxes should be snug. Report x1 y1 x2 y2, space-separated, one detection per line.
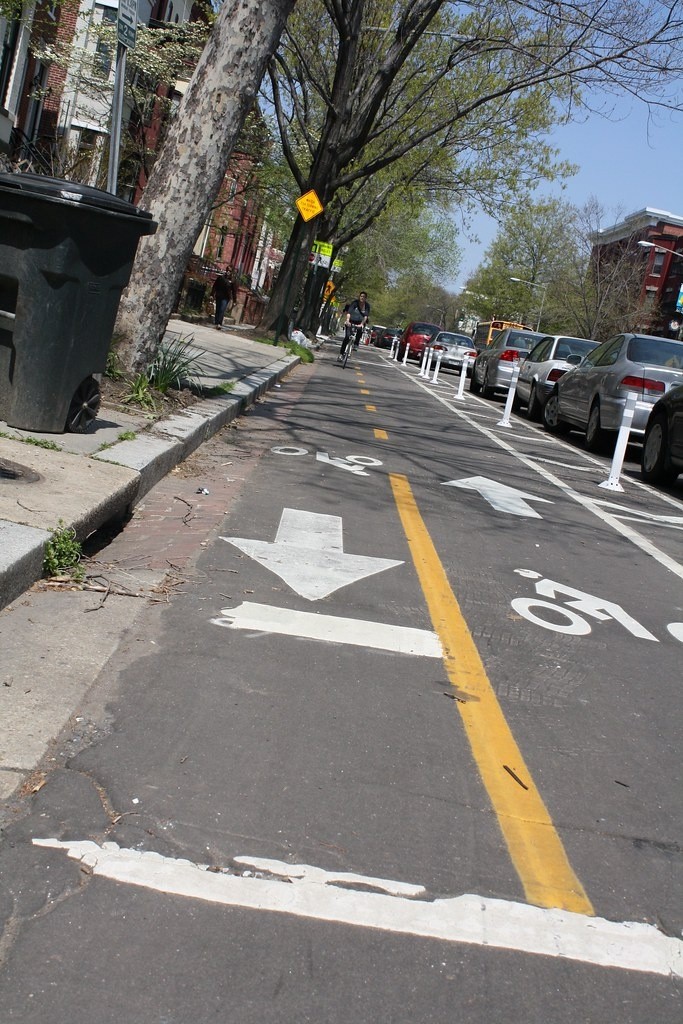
213 325 218 329
216 326 221 330
354 344 359 351
338 355 343 361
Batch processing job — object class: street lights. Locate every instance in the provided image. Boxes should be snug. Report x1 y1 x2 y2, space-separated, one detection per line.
510 277 547 332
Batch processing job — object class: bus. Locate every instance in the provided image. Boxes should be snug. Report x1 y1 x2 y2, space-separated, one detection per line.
471 320 534 355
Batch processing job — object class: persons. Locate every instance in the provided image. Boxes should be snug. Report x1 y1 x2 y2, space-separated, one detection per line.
337 292 371 362
209 265 237 330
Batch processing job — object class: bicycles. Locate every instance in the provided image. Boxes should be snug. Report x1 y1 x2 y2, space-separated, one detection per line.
342 322 365 369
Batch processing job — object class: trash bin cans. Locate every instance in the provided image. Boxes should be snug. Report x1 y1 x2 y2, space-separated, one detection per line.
0 170 159 436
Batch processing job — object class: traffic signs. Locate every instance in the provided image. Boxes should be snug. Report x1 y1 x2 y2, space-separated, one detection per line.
117 0 138 50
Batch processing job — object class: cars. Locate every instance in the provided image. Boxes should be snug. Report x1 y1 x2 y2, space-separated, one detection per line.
394 321 444 362
419 330 478 378
361 323 404 350
542 333 683 462
469 328 550 400
512 335 603 423
641 383 683 486
